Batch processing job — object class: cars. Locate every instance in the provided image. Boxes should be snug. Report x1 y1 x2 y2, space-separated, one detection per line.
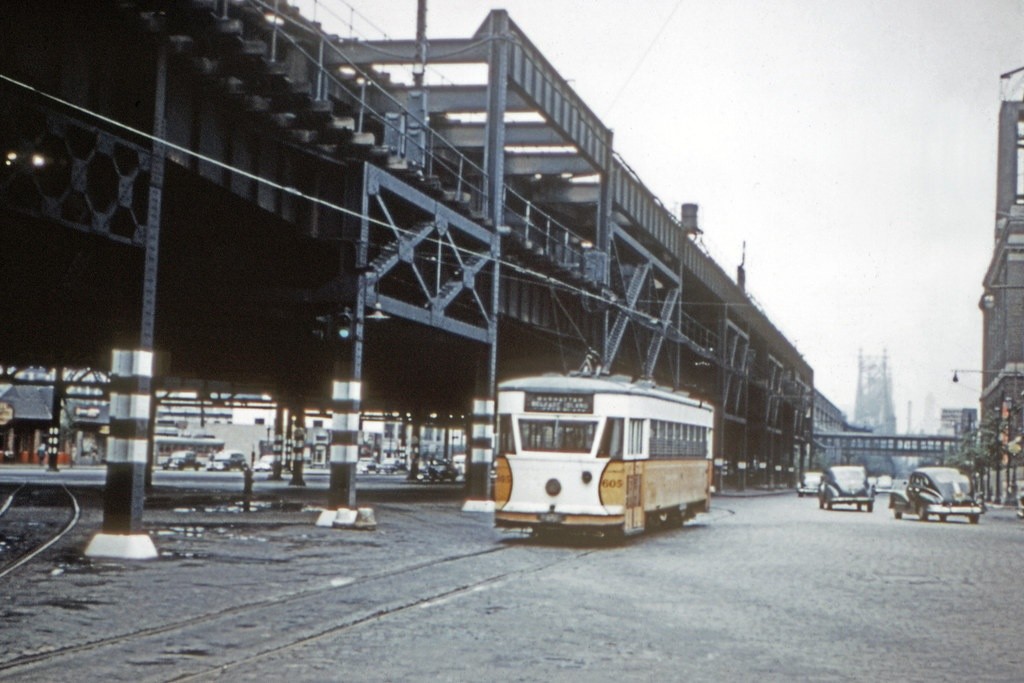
417 464 458 483
254 454 286 471
452 436 458 461
163 450 200 471
377 459 405 474
1015 485 1024 518
357 456 381 474
871 475 897 494
888 464 984 522
207 452 246 471
818 466 876 512
797 471 825 497
452 454 466 475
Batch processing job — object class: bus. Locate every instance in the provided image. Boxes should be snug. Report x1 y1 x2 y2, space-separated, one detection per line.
492 370 716 544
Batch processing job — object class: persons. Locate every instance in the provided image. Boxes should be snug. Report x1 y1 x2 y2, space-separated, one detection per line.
38 444 45 465
70 443 77 467
5 447 14 463
562 433 577 450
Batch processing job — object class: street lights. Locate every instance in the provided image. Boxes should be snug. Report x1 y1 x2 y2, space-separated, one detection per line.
952 370 1018 507
266 426 270 454
